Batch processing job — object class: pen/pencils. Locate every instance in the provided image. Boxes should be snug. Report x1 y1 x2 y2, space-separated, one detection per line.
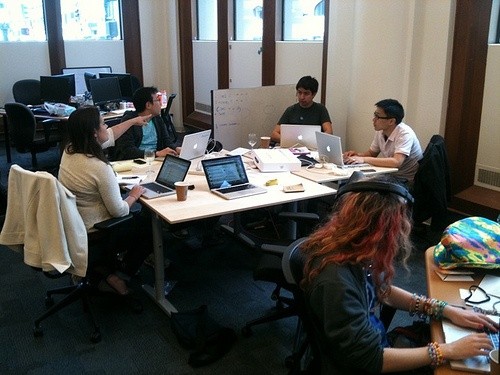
139 179 142 184
122 177 139 179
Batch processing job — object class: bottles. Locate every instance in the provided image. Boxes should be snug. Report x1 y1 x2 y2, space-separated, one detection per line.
162 92 167 106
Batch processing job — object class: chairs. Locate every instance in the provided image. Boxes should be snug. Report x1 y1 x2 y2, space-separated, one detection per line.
283 238 399 375
409 134 455 238
3 72 178 170
0 164 140 343
241 174 360 337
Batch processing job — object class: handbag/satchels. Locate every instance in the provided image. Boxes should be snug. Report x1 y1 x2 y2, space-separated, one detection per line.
44 102 76 117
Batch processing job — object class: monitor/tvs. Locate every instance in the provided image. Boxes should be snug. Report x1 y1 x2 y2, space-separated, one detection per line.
84 72 133 110
39 74 76 101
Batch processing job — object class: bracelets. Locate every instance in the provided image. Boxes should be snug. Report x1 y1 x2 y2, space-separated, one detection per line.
428 341 443 367
409 292 448 320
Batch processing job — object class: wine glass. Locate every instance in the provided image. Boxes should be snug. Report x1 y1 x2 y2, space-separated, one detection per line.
144 148 156 174
248 133 258 152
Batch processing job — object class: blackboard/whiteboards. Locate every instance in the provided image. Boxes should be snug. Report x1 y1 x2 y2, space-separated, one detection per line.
210 84 297 150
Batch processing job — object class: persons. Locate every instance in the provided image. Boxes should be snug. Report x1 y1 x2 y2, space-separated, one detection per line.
343 99 422 187
294 175 500 375
57 104 152 296
113 87 191 239
272 76 333 142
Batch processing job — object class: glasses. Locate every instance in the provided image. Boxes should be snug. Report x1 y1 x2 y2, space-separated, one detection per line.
374 112 389 121
153 97 161 102
296 91 312 96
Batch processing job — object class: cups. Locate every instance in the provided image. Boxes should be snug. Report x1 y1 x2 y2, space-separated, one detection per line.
174 182 188 201
489 349 500 375
120 102 126 109
260 136 271 148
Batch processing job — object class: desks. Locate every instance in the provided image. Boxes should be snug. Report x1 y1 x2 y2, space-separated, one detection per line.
425 244 500 374
110 146 398 317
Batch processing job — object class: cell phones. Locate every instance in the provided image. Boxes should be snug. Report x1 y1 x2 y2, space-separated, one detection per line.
134 159 146 164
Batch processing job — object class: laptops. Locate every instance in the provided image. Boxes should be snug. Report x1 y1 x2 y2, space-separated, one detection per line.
441 313 500 373
155 129 213 161
314 131 370 169
126 153 191 199
201 155 267 200
280 124 321 150
